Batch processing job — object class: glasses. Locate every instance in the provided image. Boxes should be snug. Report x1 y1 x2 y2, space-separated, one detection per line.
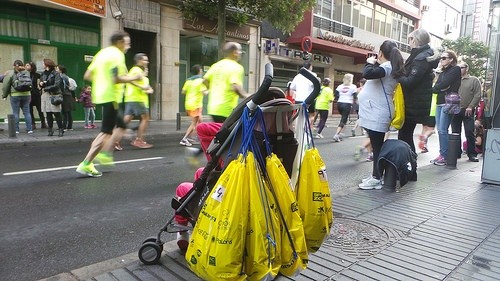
441 56 449 60
460 66 468 69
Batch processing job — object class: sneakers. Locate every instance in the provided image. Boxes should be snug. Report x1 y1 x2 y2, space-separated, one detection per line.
333 135 340 142
362 173 373 183
434 158 447 166
114 141 123 151
76 161 102 177
315 133 324 139
131 137 153 149
94 151 114 167
179 138 192 146
339 133 343 141
359 176 382 190
430 154 442 163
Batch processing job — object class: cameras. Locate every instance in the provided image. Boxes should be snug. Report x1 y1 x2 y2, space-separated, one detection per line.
367 53 377 60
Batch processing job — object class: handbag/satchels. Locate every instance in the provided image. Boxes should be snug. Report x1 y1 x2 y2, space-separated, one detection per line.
51 95 63 105
444 95 461 114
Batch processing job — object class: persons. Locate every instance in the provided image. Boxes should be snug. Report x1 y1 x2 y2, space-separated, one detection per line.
452 62 481 161
433 50 461 169
76 32 142 177
314 78 335 138
357 40 405 189
288 61 321 105
0 59 77 137
356 135 373 162
115 53 154 150
203 42 249 124
352 78 365 137
75 86 97 128
398 28 441 182
418 76 438 153
179 64 208 146
334 74 357 141
172 87 298 250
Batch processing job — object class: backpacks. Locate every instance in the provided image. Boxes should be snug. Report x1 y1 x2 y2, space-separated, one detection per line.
379 65 405 130
185 100 333 281
12 68 77 91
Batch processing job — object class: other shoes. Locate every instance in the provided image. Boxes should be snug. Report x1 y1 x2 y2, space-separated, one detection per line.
354 145 363 161
457 153 461 158
176 231 189 256
366 154 374 162
419 134 428 149
361 132 366 135
421 147 428 153
11 120 98 137
468 154 479 163
351 128 356 137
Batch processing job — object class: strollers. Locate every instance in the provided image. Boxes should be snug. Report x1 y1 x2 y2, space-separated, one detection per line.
136 62 323 266
